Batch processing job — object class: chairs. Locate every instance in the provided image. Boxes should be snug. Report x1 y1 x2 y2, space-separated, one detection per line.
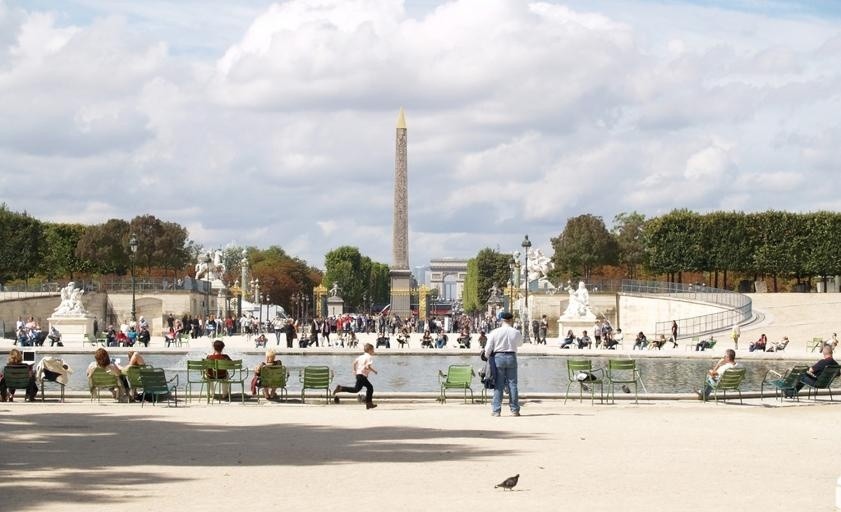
606 359 642 403
701 364 841 406
3 363 32 401
481 378 507 404
83 334 97 348
567 336 624 350
298 366 333 404
177 333 190 347
633 337 716 351
564 360 604 405
440 365 476 404
37 358 64 402
398 334 448 348
96 330 107 348
165 335 177 348
88 359 290 408
767 337 839 356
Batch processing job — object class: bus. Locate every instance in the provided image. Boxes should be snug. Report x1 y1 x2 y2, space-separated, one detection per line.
378 303 451 317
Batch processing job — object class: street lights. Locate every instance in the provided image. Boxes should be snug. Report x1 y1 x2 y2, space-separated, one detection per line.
430 295 437 320
290 291 310 325
231 248 259 303
508 256 516 328
225 292 231 319
257 294 262 333
521 234 532 343
265 295 270 325
452 303 456 320
203 253 210 316
362 295 374 335
126 234 136 322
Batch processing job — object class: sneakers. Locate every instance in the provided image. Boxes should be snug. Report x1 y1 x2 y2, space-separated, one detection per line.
334 385 339 396
366 402 377 409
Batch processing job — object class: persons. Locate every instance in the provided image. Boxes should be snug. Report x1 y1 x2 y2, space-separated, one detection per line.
203 340 233 399
573 280 589 306
577 331 592 349
483 312 523 417
166 313 548 350
332 343 379 409
12 314 61 347
101 316 150 348
696 335 716 351
560 329 576 349
633 332 646 349
647 335 666 349
750 334 767 351
122 350 146 403
593 319 623 349
671 320 678 348
86 348 133 403
783 346 841 399
770 336 789 352
254 348 282 399
697 348 746 400
731 320 741 347
819 333 838 354
0 349 39 402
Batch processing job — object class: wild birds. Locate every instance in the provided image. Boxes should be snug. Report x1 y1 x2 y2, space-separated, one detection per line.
580 383 590 393
494 472 521 492
621 384 631 394
357 392 366 403
435 395 448 403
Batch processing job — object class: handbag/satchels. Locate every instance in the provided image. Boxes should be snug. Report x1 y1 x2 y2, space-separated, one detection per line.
578 372 596 381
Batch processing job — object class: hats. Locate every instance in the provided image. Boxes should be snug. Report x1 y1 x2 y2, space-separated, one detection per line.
501 313 512 319
